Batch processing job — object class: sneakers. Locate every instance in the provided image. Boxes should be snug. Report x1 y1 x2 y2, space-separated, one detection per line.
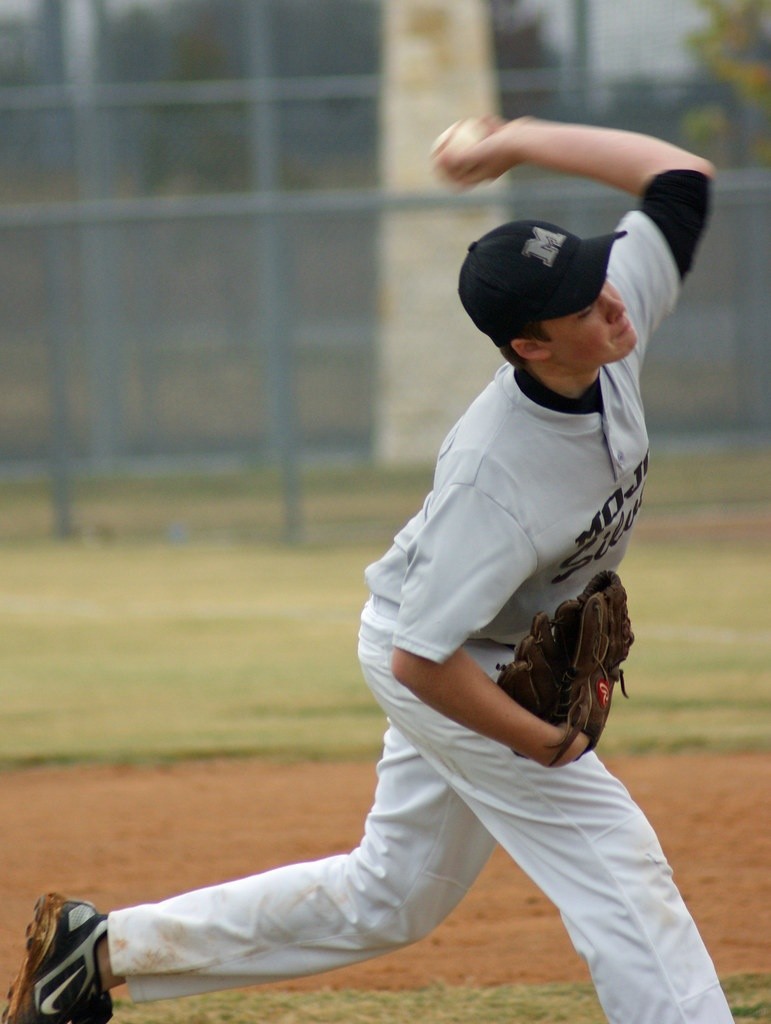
0 891 114 1024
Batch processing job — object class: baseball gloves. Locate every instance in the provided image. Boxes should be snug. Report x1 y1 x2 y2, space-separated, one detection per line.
492 569 635 767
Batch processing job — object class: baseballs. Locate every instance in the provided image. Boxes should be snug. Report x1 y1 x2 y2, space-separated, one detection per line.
436 116 489 165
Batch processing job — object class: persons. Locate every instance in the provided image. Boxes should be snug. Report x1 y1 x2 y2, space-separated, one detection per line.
0 117 736 1024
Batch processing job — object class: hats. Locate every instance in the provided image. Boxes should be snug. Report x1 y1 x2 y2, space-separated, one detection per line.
457 220 629 347
